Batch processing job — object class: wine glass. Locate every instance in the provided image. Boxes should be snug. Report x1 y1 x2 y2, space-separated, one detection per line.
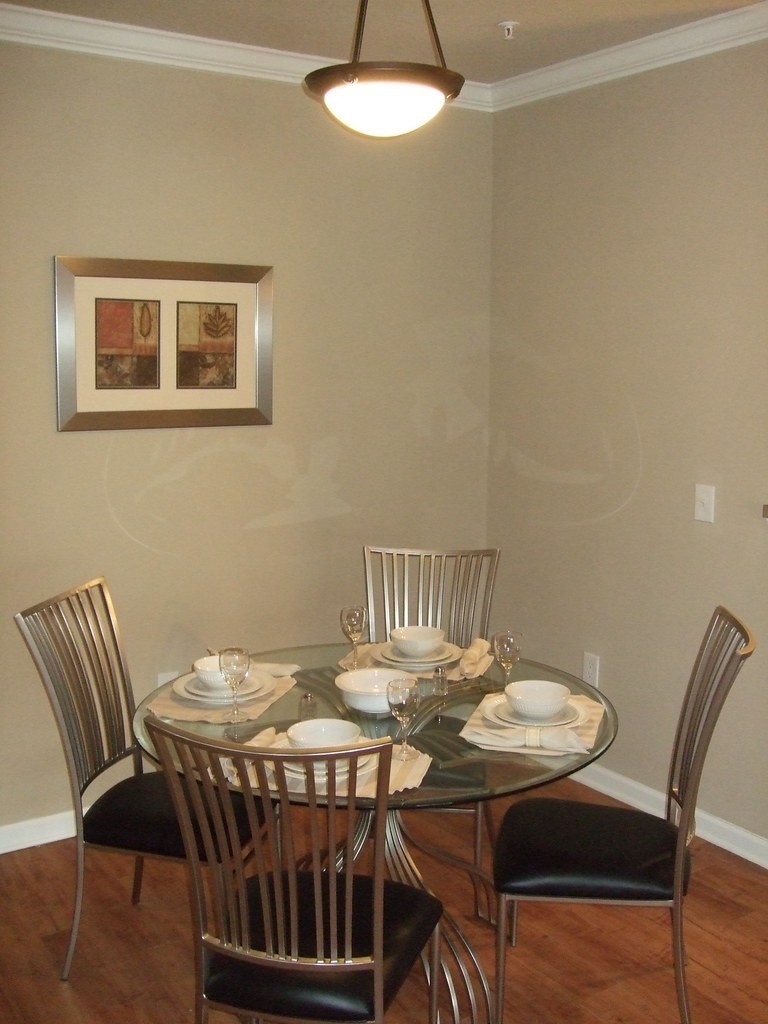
494 632 522 690
339 607 369 670
386 679 421 762
219 648 250 723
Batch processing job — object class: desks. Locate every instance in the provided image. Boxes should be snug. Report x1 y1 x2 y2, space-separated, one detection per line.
134 644 618 1024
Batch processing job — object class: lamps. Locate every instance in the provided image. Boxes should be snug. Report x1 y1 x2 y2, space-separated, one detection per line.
304 0 465 138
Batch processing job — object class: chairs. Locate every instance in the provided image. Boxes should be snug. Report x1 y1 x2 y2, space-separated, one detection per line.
13 576 282 982
144 715 443 1024
364 545 497 919
494 606 756 1024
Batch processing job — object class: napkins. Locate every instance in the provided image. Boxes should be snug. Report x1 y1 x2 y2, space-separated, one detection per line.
460 638 495 679
466 726 591 754
206 727 276 784
207 647 301 676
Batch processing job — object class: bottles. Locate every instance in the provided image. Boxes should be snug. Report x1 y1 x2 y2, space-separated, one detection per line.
432 664 449 696
298 691 317 722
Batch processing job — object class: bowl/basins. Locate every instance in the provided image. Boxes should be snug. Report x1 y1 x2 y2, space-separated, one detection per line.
390 627 445 657
505 680 570 719
193 656 250 694
287 718 361 749
334 668 417 713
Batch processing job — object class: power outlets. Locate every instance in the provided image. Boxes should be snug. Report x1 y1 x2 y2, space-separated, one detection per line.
582 652 599 688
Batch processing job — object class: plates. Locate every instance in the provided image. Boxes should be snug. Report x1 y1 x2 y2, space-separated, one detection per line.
370 642 463 672
263 738 379 782
381 642 453 663
480 698 590 730
184 673 262 696
172 670 276 702
282 737 371 775
494 701 579 726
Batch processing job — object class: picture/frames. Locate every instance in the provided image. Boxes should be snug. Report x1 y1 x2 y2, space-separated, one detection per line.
54 256 274 433
